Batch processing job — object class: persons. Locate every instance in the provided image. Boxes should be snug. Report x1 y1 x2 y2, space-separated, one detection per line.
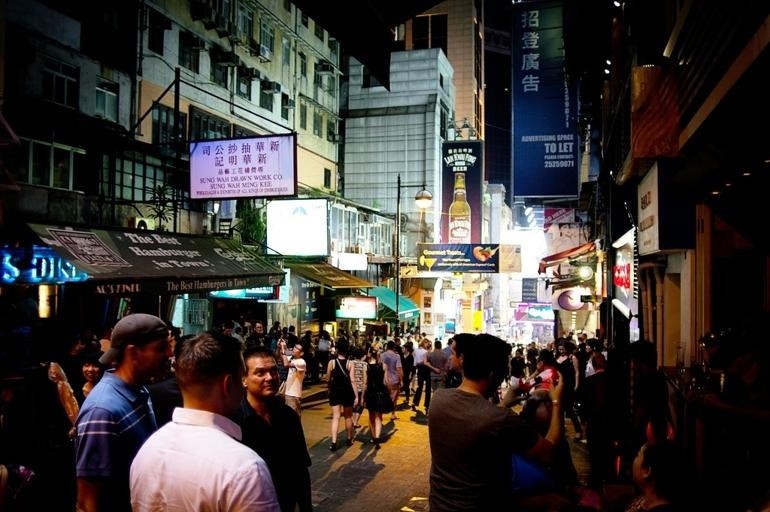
624 442 681 510
326 319 670 451
235 346 315 511
128 328 281 511
427 333 564 510
73 314 175 511
45 319 333 424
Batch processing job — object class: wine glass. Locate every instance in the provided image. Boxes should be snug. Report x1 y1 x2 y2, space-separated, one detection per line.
422 258 438 271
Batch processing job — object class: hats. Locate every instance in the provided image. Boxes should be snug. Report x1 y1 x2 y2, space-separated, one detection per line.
98 313 168 364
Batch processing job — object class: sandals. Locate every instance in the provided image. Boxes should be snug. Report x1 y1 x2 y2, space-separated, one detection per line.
329 424 380 450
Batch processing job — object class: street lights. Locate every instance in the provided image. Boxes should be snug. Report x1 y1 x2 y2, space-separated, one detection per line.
394 176 434 343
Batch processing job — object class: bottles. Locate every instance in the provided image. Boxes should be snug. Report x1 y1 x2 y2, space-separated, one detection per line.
448 172 472 244
